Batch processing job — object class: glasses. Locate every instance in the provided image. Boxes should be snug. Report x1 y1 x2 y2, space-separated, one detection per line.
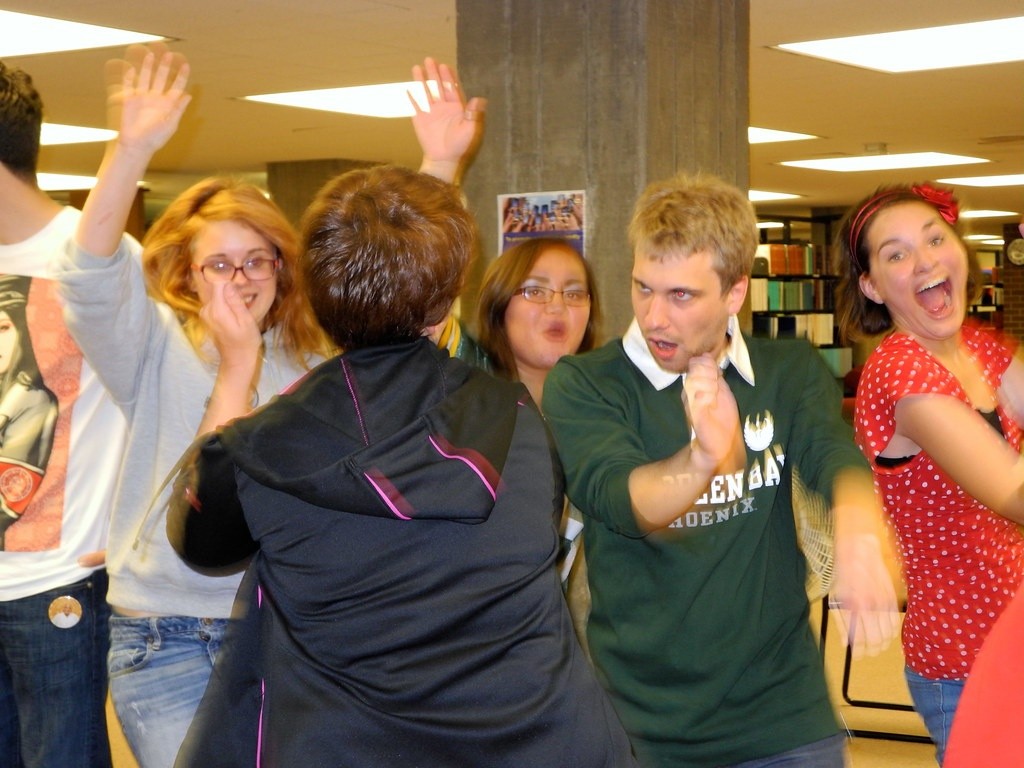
513 286 590 307
191 258 278 284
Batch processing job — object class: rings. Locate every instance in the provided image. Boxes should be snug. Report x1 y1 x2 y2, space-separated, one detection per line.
465 109 479 122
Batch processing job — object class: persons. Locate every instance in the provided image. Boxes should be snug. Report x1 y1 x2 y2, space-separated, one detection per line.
166 167 642 768
49 55 487 768
0 67 151 767
402 60 604 605
543 167 900 766
834 180 1024 768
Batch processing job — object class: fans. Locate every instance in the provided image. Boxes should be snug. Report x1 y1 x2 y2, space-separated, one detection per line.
791 468 838 601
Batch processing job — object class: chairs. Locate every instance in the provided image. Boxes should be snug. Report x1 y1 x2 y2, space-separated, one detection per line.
821 591 933 744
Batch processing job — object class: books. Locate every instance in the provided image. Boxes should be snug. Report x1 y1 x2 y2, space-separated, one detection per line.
747 243 1004 397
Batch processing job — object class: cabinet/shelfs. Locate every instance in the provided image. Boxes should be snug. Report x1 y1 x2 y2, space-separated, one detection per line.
751 213 858 398
